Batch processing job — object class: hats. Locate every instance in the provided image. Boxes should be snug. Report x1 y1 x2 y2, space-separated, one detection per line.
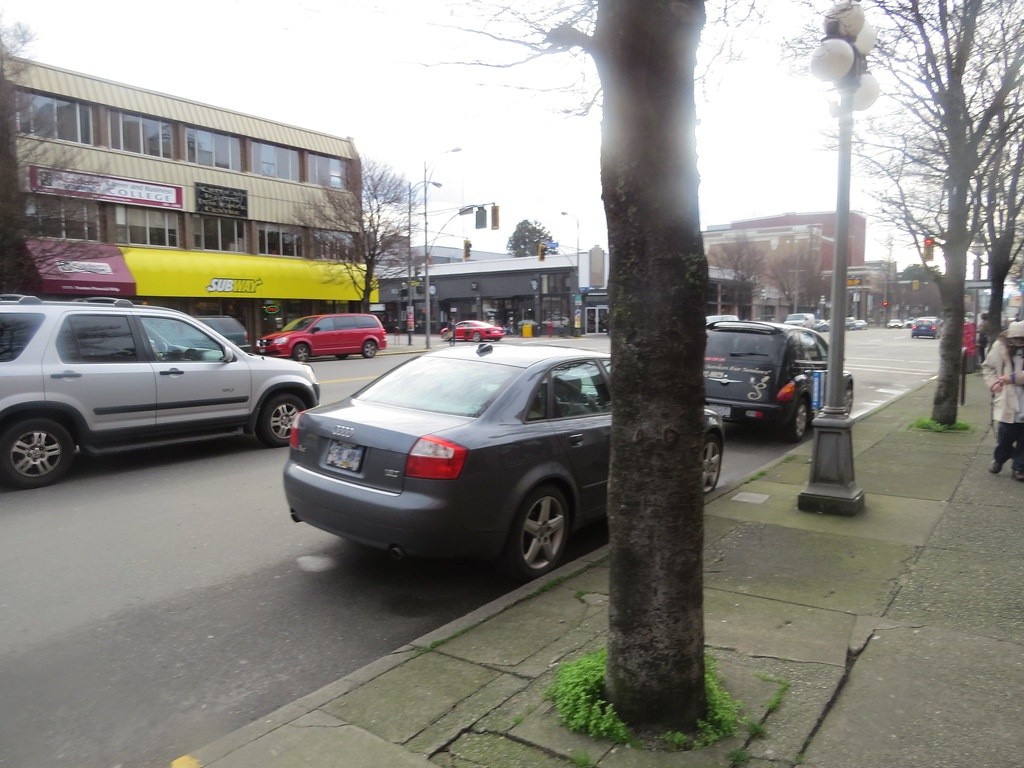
1007 321 1024 339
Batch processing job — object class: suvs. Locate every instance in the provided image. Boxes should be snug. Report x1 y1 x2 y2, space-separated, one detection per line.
0 291 320 489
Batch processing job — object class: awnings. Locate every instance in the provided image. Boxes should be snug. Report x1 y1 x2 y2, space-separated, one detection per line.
119 247 379 303
16 238 137 295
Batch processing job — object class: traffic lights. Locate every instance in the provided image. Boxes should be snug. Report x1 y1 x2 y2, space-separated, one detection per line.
883 298 888 308
539 243 547 262
463 238 472 261
923 238 936 266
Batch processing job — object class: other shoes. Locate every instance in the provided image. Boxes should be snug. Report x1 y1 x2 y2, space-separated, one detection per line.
989 460 1002 474
1013 471 1024 481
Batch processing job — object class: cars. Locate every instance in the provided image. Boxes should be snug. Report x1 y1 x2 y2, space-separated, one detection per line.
705 314 738 328
438 319 506 344
282 339 726 586
784 313 868 331
703 317 856 444
886 317 943 340
154 313 254 357
254 312 387 362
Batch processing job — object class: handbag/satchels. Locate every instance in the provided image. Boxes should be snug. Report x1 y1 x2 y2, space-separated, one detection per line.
993 384 1020 413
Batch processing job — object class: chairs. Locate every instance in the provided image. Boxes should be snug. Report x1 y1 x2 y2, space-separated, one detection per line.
553 373 581 401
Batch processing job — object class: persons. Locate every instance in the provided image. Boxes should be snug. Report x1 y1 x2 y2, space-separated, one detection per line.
504 320 514 336
977 314 989 365
1014 314 1021 321
447 317 456 346
982 320 1024 481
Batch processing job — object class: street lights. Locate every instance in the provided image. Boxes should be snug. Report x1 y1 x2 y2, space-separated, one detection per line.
796 2 883 515
423 145 464 349
560 212 582 338
407 178 444 347
